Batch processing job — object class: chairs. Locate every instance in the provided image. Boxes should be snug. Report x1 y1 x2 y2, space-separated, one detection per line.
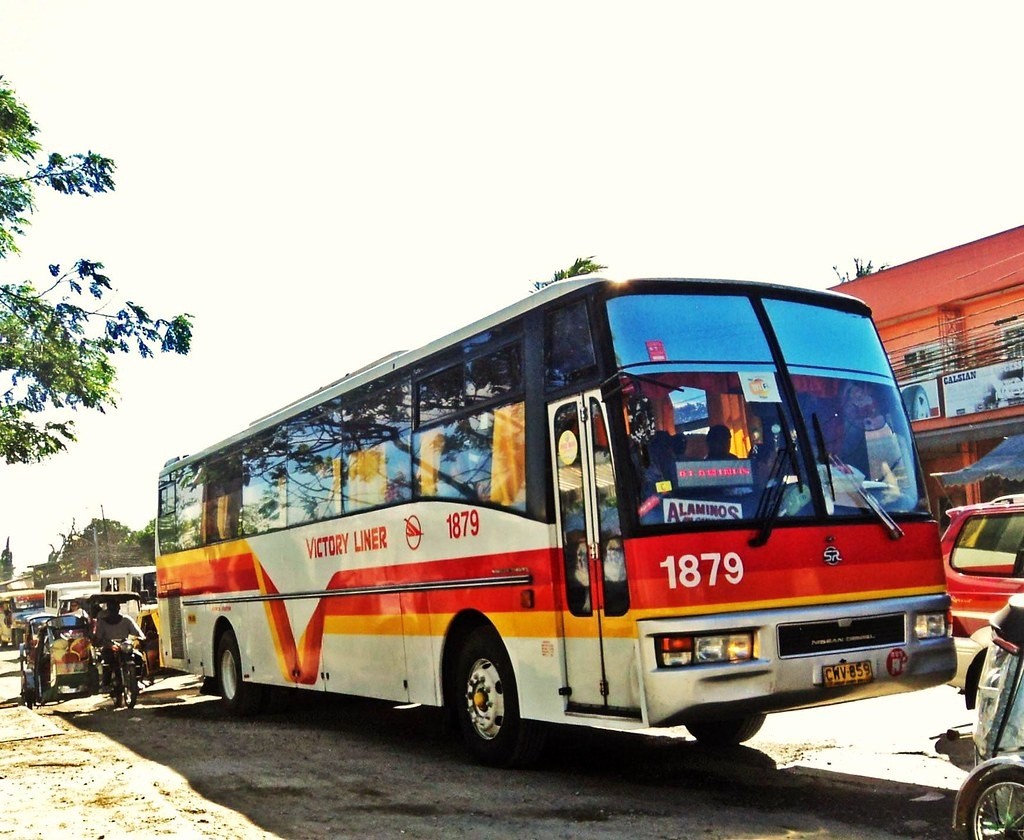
683 434 710 460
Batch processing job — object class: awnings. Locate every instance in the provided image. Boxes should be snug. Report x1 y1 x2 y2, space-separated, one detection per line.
941 434 1024 487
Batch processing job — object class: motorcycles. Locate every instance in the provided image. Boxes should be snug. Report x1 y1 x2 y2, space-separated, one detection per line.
19 591 147 709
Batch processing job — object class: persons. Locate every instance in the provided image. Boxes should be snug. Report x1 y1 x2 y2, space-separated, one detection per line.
704 424 739 461
95 600 146 692
61 601 88 618
725 444 789 496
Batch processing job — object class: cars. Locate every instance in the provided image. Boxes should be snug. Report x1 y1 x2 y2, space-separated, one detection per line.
942 493 1024 709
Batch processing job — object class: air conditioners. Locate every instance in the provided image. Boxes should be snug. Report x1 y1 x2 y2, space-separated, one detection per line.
902 349 926 367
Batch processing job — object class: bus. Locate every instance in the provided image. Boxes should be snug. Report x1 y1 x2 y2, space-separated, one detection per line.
44 581 100 615
98 566 156 622
0 590 46 650
154 276 957 768
